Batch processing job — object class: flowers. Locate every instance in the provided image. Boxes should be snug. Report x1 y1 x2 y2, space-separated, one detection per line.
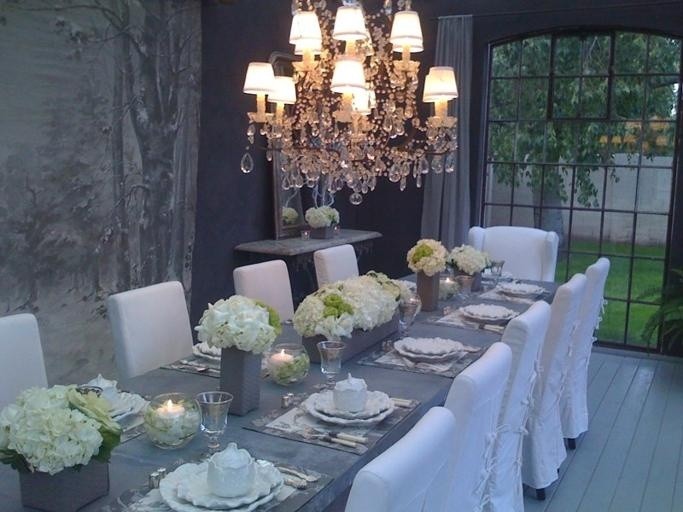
282 207 298 226
306 206 340 228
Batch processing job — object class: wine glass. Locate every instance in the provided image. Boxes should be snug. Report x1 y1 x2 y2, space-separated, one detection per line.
399 294 421 336
317 341 346 390
196 391 234 461
75 386 103 398
482 261 504 295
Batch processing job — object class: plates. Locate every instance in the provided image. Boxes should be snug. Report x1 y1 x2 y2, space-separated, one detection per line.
306 390 397 425
394 279 416 289
496 281 545 295
459 303 519 321
160 458 284 512
394 337 463 362
192 341 222 362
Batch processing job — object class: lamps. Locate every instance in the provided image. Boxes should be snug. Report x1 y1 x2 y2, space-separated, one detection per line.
240 1 459 205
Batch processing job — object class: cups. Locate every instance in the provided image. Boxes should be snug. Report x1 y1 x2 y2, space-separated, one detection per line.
333 373 369 413
207 442 256 498
301 230 311 241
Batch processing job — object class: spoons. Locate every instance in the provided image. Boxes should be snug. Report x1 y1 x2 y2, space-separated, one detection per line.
171 363 208 372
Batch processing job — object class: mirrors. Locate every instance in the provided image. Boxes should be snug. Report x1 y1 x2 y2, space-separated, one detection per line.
269 52 306 241
